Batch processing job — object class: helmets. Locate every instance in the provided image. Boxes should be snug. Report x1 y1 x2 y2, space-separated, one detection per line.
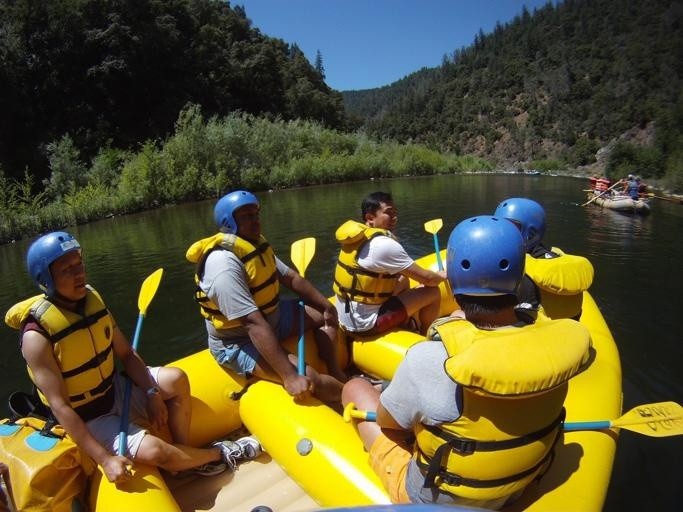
214 192 260 235
450 216 525 295
492 198 545 252
27 232 81 294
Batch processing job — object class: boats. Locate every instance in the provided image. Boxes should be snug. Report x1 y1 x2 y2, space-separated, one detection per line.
0 241 683 512
587 180 650 216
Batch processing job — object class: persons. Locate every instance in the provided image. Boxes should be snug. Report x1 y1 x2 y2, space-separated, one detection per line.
337 192 448 337
494 197 594 323
340 215 591 512
186 190 348 403
21 231 262 485
610 174 649 201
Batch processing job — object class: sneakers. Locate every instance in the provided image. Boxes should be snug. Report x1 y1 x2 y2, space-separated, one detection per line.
210 438 261 463
174 462 226 477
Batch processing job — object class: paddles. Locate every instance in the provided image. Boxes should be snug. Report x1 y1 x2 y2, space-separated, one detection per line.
290 237 315 393
342 402 682 437
116 268 163 477
581 179 623 207
424 219 443 272
638 192 655 199
